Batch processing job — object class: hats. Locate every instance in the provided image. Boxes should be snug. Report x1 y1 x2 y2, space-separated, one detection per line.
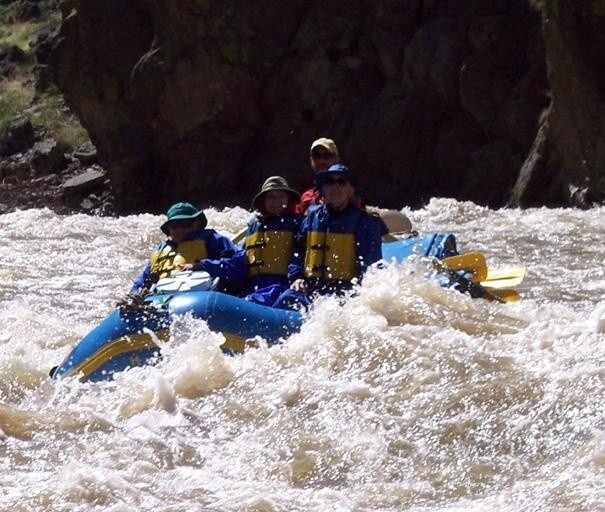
253 175 301 212
316 163 365 198
310 138 338 154
160 202 207 236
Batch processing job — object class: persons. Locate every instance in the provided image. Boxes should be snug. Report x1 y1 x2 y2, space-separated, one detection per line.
271 163 383 312
293 137 366 217
124 202 250 297
236 175 305 307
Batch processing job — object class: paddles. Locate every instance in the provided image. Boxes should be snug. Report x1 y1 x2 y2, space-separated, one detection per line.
438 253 486 282
477 268 524 288
486 287 520 303
